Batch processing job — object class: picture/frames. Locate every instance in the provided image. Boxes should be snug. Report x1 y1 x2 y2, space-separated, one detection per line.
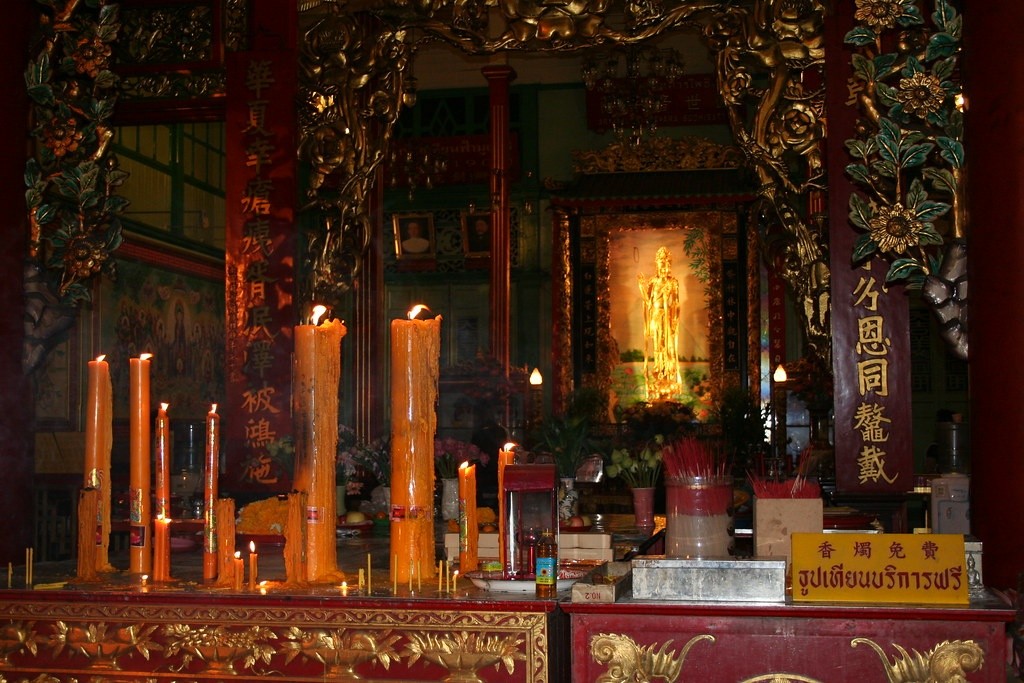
392 212 437 261
69 208 228 434
460 211 491 259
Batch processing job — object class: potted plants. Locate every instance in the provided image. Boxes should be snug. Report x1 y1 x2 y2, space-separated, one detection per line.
545 378 608 519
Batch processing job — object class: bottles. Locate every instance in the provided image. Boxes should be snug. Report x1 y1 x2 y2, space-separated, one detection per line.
535 533 558 599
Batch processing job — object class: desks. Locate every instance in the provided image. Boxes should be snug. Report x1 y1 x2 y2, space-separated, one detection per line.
0 514 1024 683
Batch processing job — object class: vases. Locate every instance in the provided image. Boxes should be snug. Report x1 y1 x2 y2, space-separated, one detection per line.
628 486 653 527
371 483 390 507
441 479 460 520
336 486 346 517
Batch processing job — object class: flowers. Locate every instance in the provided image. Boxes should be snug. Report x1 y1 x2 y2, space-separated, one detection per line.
435 436 490 478
336 425 391 492
606 434 674 489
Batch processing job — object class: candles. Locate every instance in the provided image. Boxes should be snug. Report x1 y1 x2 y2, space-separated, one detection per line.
154 512 171 583
367 554 371 594
26 548 29 584
447 565 450 593
29 548 33 584
285 306 340 588
393 553 398 595
78 356 108 579
498 443 516 570
409 559 413 591
418 560 421 593
771 365 787 482
249 540 257 587
439 560 442 590
458 461 477 573
8 562 12 587
529 369 544 463
453 570 459 590
155 402 171 520
388 304 441 586
202 404 241 592
128 354 152 575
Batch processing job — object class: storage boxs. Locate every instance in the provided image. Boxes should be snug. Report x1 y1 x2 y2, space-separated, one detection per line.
752 499 824 562
631 555 787 604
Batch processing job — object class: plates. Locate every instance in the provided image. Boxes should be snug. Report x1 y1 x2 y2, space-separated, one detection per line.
236 533 287 544
562 526 592 531
465 568 590 592
152 537 195 551
